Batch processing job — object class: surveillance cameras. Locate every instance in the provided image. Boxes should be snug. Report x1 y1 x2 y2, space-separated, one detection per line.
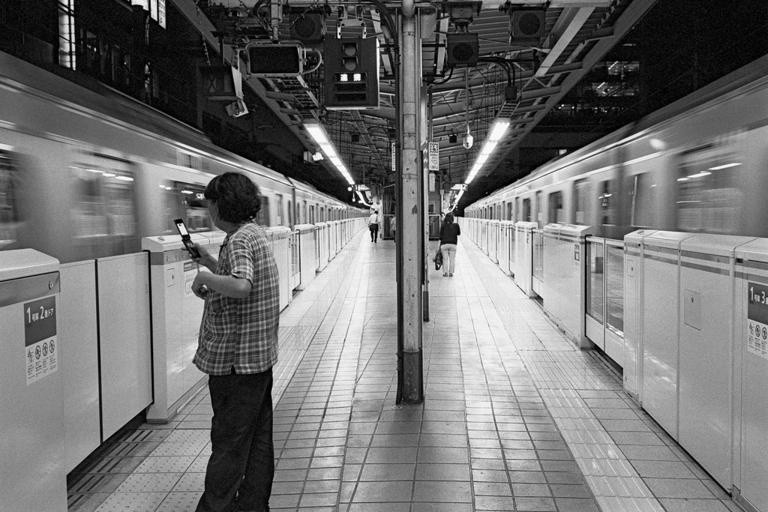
462 136 474 150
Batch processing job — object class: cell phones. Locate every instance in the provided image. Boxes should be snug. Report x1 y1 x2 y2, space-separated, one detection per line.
174 218 201 258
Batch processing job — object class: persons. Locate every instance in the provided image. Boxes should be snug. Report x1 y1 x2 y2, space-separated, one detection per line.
391 216 396 243
437 212 461 277
173 171 279 512
367 211 381 243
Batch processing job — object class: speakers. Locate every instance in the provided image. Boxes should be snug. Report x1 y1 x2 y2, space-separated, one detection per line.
510 8 545 46
292 12 325 41
446 32 480 66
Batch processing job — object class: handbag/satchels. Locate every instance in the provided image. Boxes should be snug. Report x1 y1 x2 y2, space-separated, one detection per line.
434 249 444 271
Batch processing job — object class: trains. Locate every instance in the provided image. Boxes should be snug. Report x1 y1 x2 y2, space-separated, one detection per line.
0 47 371 264
464 54 768 240
